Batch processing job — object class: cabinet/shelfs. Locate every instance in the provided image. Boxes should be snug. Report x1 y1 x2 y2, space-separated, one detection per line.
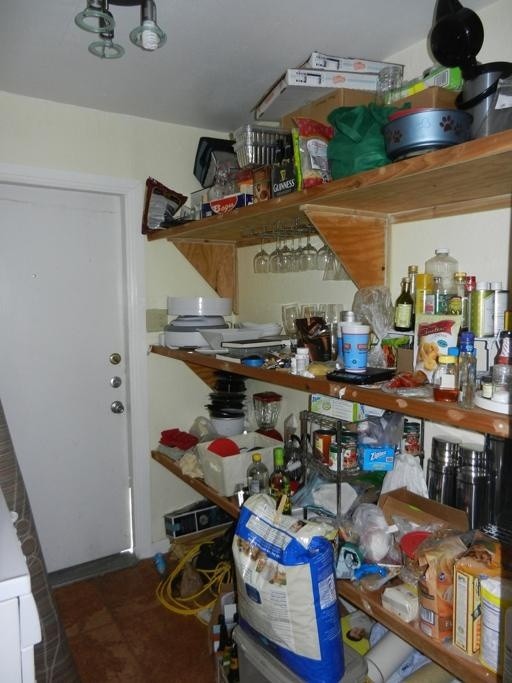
147 132 512 682
298 410 402 531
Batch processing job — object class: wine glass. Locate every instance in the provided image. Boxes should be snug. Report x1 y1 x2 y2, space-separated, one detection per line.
253 224 352 281
281 304 342 360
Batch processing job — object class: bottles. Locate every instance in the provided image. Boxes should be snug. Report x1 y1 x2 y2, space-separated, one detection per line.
375 65 403 105
290 348 309 375
247 448 291 515
213 614 239 683
433 331 477 410
394 247 508 337
494 329 512 364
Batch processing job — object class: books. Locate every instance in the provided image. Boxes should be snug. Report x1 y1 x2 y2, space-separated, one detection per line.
220 338 282 349
215 353 245 364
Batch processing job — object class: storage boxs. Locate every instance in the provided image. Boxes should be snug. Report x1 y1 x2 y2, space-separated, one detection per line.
280 88 376 135
231 625 369 682
195 432 286 497
388 86 460 110
376 487 470 538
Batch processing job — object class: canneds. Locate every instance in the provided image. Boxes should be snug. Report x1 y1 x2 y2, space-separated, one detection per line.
495 290 509 337
313 418 421 473
470 289 494 337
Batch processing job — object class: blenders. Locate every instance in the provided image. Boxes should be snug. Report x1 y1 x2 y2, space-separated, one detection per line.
252 392 283 442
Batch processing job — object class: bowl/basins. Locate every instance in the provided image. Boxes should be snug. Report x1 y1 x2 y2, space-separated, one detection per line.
196 321 282 351
379 109 474 163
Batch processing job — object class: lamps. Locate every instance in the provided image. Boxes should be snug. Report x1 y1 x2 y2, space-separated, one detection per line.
75 0 166 60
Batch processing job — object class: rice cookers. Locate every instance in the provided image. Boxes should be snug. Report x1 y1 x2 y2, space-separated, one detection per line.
430 0 512 138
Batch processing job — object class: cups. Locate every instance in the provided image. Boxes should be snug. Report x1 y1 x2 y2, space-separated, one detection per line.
337 321 370 373
492 364 512 403
